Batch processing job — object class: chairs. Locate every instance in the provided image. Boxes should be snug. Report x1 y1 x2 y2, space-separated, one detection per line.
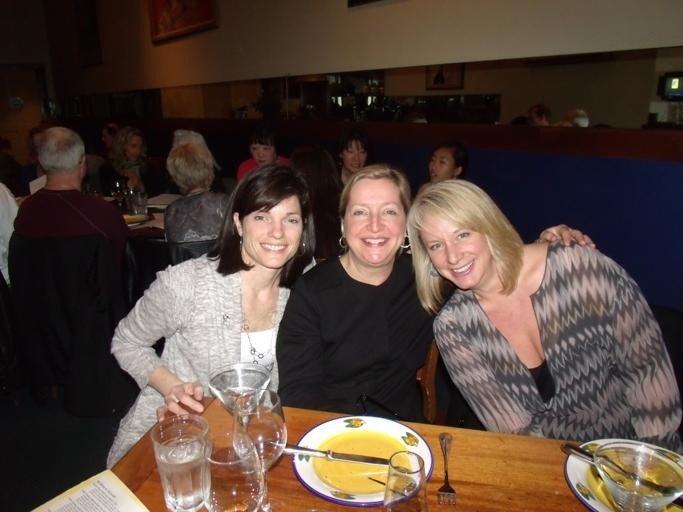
417 333 451 421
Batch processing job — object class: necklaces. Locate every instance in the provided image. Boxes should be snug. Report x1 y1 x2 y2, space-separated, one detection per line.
243 293 282 366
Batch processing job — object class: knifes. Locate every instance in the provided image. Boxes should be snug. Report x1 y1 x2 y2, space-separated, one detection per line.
266 440 389 465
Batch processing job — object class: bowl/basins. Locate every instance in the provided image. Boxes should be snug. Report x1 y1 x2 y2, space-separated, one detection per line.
593 442 683 512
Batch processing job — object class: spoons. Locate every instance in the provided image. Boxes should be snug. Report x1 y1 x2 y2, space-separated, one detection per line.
560 443 683 507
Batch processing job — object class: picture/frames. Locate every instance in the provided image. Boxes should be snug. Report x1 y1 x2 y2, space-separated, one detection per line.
425 69 463 92
147 0 220 41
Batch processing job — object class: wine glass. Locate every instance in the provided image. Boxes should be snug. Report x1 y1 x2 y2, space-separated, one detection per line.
110 177 127 209
202 431 264 512
232 390 289 512
127 186 139 201
207 361 272 432
384 451 428 512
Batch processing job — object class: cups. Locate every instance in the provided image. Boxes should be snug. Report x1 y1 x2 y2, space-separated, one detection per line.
150 413 211 512
131 191 149 215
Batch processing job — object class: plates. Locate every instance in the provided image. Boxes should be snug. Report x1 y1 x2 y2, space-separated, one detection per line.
145 204 167 210
566 438 683 512
127 222 141 227
292 415 433 507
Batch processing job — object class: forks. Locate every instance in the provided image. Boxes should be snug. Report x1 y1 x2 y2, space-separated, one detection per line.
436 432 456 506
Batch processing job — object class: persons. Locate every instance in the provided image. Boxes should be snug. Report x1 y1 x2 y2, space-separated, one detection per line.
105 162 318 471
1 120 469 286
526 101 591 126
405 179 682 458
276 164 597 421
